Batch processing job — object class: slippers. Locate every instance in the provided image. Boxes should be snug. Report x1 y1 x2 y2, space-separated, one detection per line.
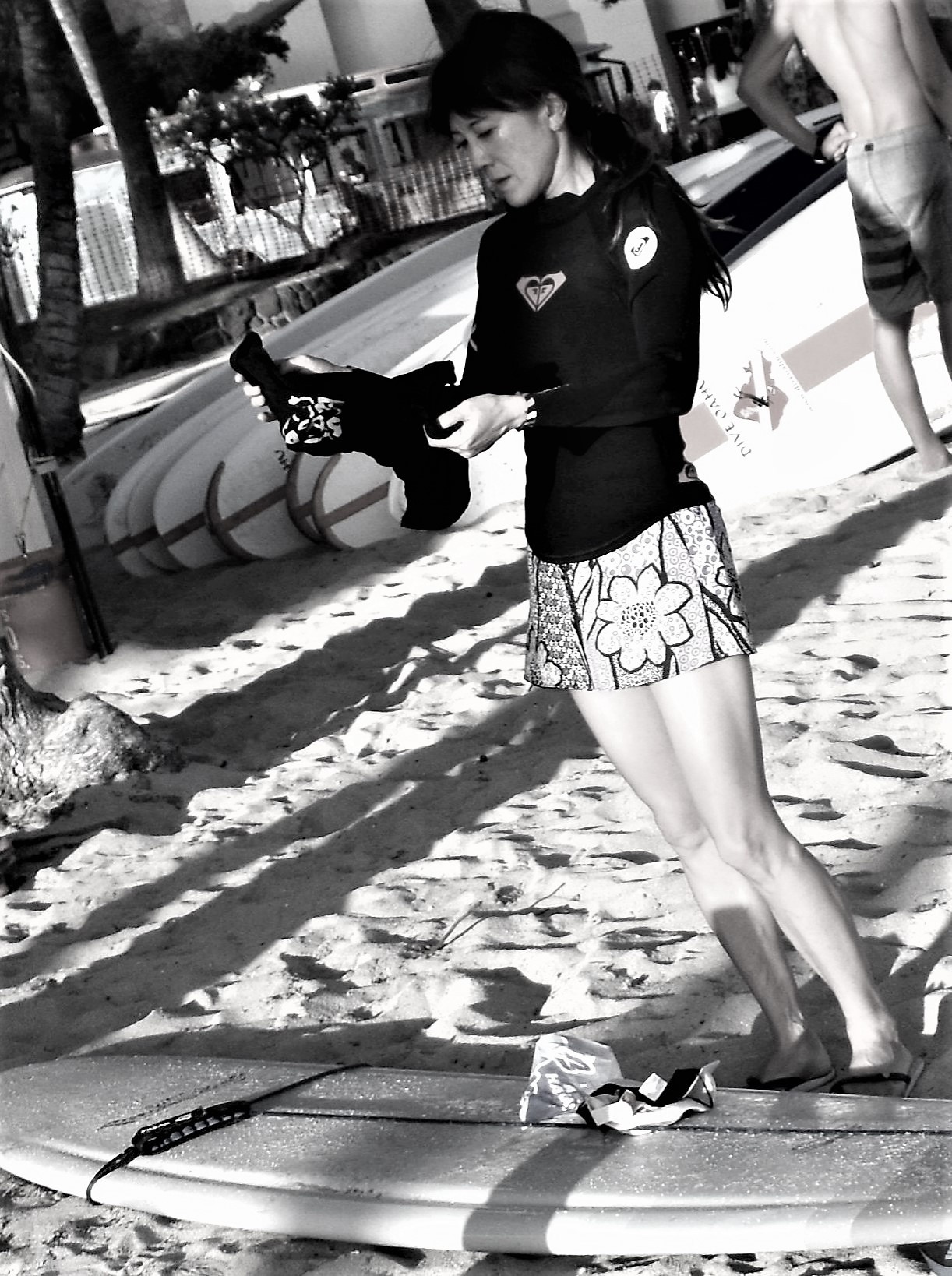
741 1062 838 1093
828 1050 926 1097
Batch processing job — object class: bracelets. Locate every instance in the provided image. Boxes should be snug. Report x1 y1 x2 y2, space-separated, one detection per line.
513 391 539 431
812 135 827 166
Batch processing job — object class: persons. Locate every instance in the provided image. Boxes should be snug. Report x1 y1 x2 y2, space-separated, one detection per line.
645 79 691 164
734 0 952 478
338 146 391 233
229 8 915 1103
687 28 766 149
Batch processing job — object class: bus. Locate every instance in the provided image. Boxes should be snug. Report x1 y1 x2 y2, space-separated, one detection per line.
0 43 633 338
